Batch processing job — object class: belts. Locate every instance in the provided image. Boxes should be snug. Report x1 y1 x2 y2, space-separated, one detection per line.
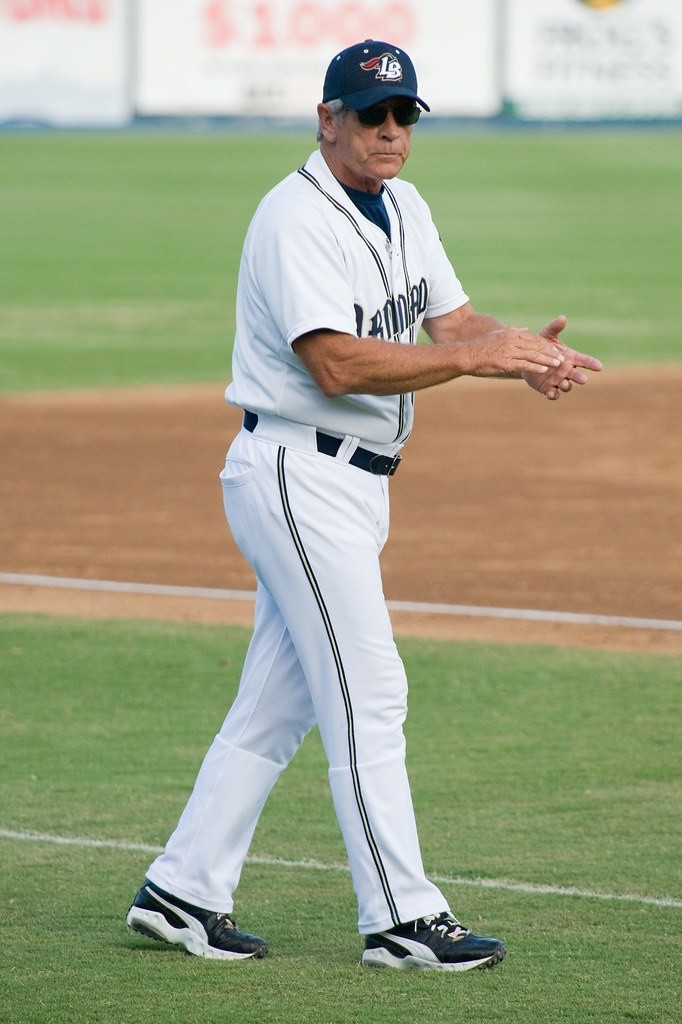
243 410 403 478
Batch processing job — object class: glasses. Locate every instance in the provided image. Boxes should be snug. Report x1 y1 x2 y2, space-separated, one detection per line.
351 104 421 126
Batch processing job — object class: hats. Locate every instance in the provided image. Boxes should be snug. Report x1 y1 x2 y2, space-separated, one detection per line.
324 38 430 114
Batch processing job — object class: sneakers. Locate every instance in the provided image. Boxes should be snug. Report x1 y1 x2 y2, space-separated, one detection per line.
363 911 506 972
127 877 269 960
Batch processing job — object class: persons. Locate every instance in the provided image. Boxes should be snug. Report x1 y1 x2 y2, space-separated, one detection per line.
127 36 606 970
